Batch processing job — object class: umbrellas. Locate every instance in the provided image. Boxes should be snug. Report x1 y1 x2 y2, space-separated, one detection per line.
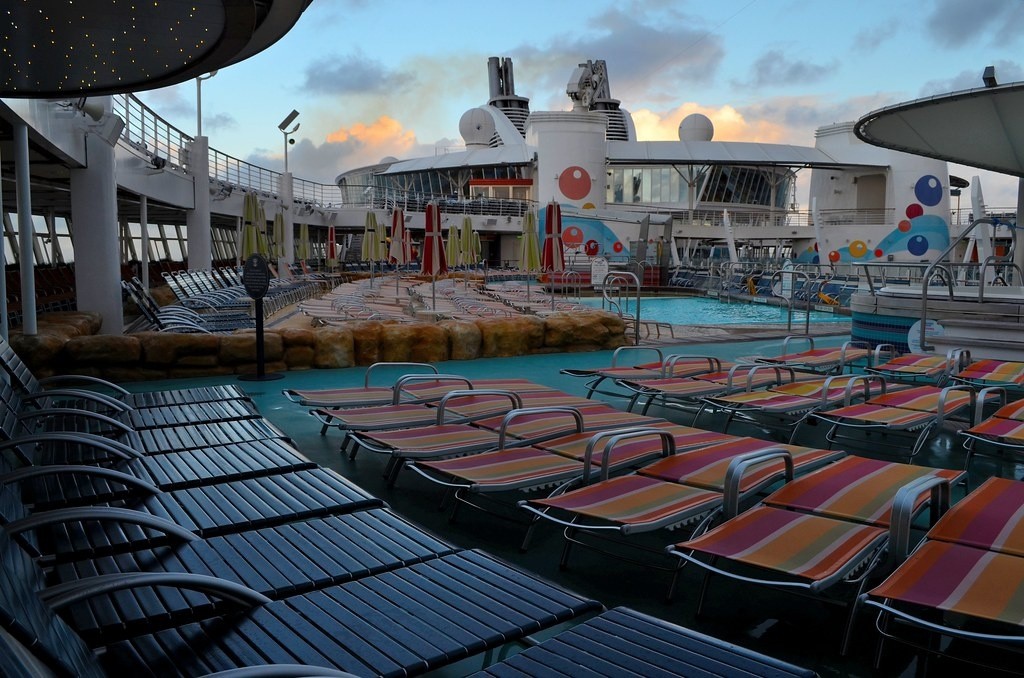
239 187 482 314
518 210 543 303
541 196 567 311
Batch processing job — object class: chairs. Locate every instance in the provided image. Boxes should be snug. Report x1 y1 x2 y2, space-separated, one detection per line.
0 255 1024 678
